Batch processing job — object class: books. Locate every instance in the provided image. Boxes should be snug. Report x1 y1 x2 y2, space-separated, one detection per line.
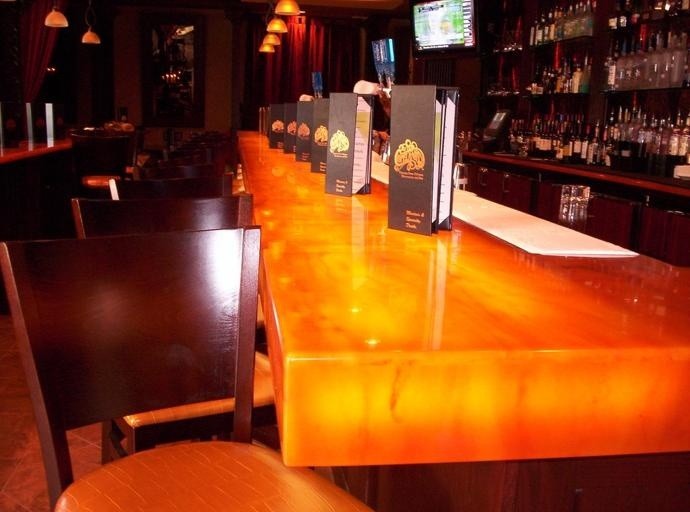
258 106 270 136
269 104 284 149
296 101 313 162
284 102 297 154
311 98 328 174
388 84 460 236
325 93 374 197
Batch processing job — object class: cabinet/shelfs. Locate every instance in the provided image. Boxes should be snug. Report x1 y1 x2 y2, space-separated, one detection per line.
463 1 690 182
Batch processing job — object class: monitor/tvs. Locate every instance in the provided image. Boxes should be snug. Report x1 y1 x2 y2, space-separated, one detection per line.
484 109 512 136
409 0 481 60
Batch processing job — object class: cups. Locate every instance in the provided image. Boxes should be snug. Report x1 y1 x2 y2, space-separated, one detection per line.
559 207 587 225
561 185 591 207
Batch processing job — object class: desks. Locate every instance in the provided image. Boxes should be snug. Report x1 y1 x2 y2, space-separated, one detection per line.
235 129 688 511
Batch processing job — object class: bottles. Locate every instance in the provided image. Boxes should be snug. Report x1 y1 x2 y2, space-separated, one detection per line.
508 0 690 178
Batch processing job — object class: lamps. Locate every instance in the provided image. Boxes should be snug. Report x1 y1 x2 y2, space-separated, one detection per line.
44 0 69 29
259 1 299 53
80 14 103 44
